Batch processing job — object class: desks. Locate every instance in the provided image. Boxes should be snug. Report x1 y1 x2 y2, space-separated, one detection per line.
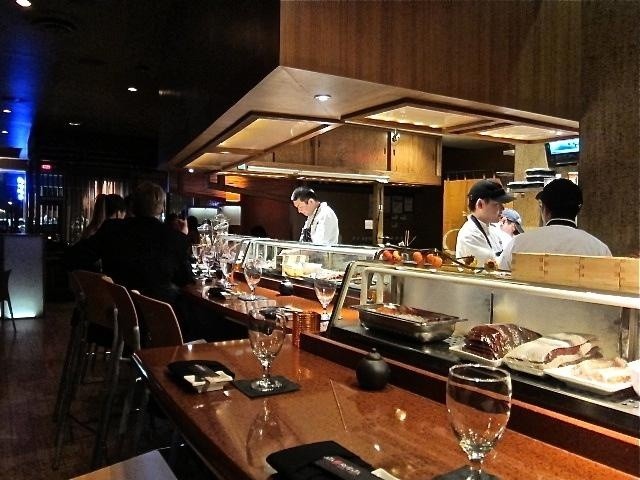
132 337 638 478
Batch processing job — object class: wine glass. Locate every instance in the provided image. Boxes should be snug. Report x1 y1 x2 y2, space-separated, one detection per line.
248 308 287 392
218 253 236 289
200 248 218 275
444 363 513 480
241 255 264 300
312 275 339 322
192 242 205 270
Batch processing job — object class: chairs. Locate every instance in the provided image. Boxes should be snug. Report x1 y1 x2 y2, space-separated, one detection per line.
50 267 206 470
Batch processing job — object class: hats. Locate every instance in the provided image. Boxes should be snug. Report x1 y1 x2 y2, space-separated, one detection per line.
469 180 516 203
502 207 524 233
536 178 583 212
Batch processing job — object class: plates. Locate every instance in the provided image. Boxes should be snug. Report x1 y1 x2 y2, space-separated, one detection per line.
542 356 634 396
447 343 504 368
501 356 544 378
183 370 235 394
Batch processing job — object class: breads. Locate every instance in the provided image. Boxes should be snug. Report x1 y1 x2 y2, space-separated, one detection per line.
376 303 424 315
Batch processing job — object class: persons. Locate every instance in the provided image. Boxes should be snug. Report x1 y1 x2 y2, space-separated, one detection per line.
184 216 203 248
121 194 135 216
97 181 200 343
289 185 339 246
165 213 180 232
76 194 109 242
455 178 513 269
494 206 526 238
69 194 126 348
498 178 624 361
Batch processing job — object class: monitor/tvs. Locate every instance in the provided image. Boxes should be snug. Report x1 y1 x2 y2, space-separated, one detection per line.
544 137 579 168
